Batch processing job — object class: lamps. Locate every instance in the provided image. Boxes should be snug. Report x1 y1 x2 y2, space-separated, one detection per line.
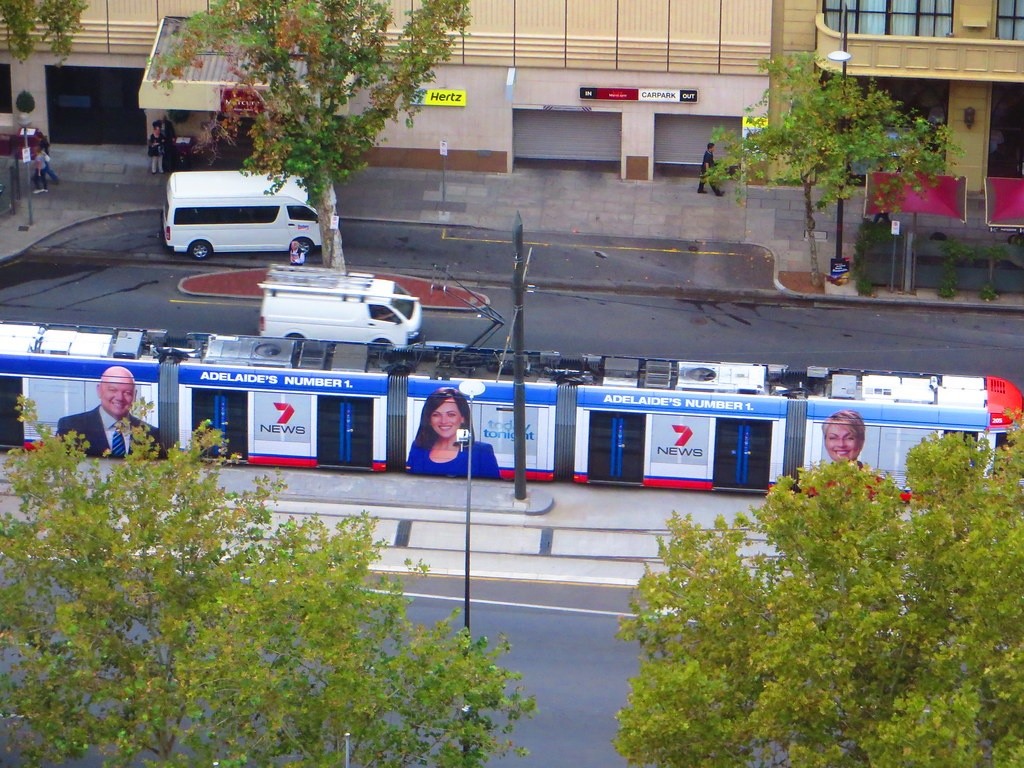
964 107 975 130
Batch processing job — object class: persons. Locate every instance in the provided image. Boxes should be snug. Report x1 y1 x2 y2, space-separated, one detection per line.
809 410 884 501
147 113 175 174
873 212 889 222
405 387 499 478
32 132 59 193
290 241 305 266
57 366 159 458
698 143 724 196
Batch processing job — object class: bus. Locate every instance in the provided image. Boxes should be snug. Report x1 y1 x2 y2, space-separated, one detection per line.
0 320 1024 504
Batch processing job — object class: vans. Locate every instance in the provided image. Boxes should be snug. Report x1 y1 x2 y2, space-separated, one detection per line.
161 168 323 260
258 263 423 348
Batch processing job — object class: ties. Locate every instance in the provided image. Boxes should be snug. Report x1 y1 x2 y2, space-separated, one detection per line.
111 422 126 457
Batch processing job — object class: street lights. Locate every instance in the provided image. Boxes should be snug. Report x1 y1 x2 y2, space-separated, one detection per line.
828 2 851 282
456 379 488 753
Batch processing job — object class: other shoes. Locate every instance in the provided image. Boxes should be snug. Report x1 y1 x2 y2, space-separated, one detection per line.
33 189 40 193
40 188 48 193
716 191 725 196
698 190 708 193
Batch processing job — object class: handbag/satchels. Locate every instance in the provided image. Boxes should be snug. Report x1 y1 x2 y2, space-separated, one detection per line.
41 150 51 162
148 145 159 156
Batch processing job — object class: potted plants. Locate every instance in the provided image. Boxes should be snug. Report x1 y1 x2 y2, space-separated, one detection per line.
16 89 36 128
